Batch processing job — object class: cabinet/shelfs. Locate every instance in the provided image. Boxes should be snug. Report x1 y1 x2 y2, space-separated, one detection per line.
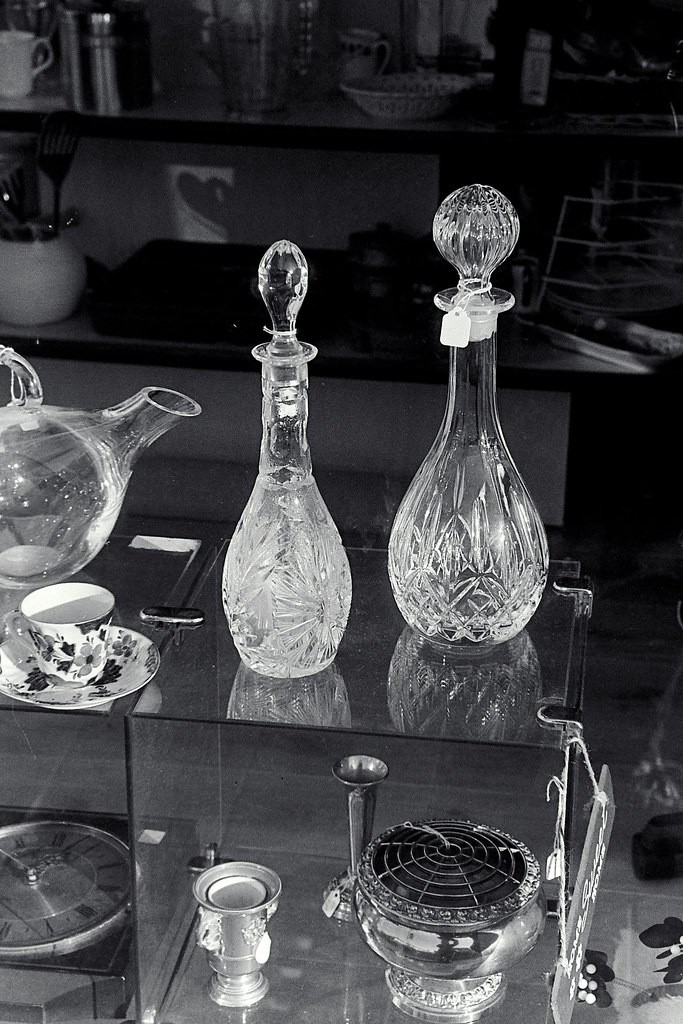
0 80 655 1024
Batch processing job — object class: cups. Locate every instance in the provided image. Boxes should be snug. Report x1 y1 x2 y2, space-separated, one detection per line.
0 31 54 100
60 6 157 112
335 28 391 82
6 582 116 689
211 0 301 123
192 861 283 1008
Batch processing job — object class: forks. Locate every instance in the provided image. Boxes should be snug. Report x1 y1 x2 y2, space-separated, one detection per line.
0 121 83 242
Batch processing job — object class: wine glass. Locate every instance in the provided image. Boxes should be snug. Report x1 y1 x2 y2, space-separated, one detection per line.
3 2 63 101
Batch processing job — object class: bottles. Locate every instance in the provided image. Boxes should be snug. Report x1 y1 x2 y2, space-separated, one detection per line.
222 239 352 678
387 183 550 649
0 223 87 327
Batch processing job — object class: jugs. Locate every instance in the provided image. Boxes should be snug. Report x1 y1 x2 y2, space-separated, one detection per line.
0 344 203 590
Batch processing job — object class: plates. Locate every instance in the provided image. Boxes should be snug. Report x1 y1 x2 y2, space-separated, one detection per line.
336 72 478 121
0 625 161 710
